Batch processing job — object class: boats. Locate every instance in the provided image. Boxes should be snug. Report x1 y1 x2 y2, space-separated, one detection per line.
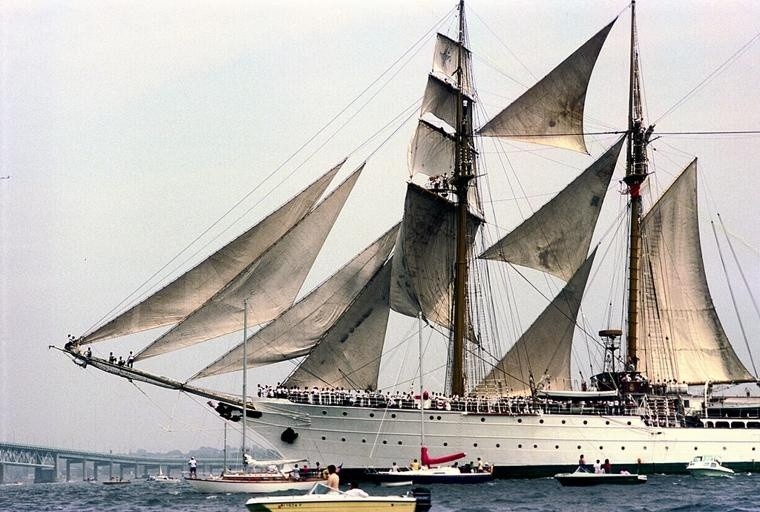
550 463 651 490
684 452 740 481
5 480 25 488
82 470 181 487
535 383 620 400
241 480 438 512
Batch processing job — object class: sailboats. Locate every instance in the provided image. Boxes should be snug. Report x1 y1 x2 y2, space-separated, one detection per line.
179 299 341 492
39 2 760 481
363 311 500 486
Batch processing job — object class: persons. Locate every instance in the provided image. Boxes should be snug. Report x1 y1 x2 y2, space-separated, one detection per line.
126 350 136 368
314 461 322 479
346 482 352 490
617 354 623 372
391 461 402 472
598 464 606 473
323 465 340 495
603 458 612 474
342 480 371 496
605 354 615 371
285 463 301 480
116 356 126 366
189 456 197 479
466 460 475 472
422 372 687 426
410 458 421 469
579 454 586 472
483 462 490 473
620 465 632 474
258 382 417 409
476 457 483 473
109 352 117 364
593 459 601 473
84 347 92 358
302 465 309 478
454 461 459 467
